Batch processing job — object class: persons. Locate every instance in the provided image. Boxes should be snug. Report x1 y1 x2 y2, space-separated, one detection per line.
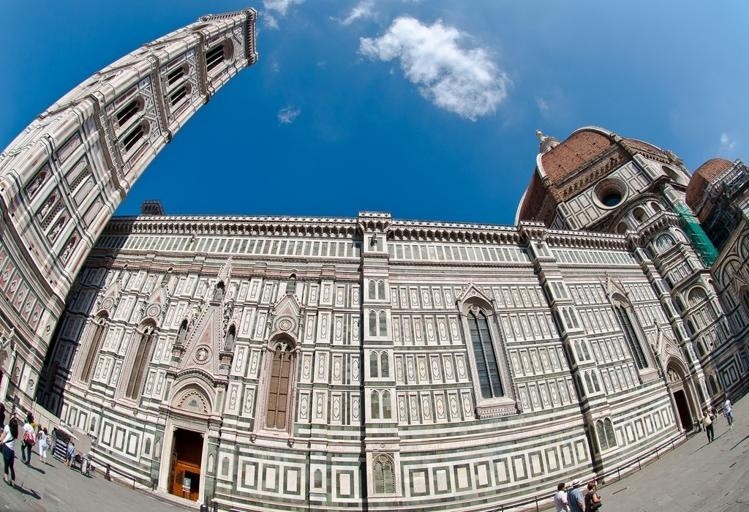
585 480 603 512
567 479 585 512
702 395 735 444
0 402 111 487
553 482 570 512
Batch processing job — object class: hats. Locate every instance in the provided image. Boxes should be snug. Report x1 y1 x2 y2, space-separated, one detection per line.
569 478 580 486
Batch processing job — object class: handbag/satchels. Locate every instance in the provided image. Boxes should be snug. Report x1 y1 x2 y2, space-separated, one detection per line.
591 502 601 509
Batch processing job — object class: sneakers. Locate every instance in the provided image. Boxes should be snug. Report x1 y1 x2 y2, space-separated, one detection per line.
25 461 31 466
10 480 15 486
3 478 10 484
20 459 26 464
